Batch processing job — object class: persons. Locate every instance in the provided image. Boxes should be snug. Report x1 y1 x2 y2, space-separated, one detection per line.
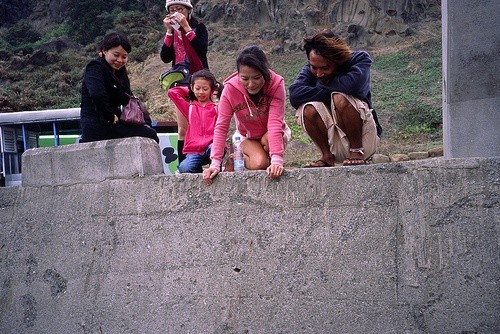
160 0 208 173
79 32 159 143
168 69 224 174
289 30 382 167
202 45 291 185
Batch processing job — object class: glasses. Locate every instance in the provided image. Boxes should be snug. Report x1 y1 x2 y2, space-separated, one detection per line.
168 6 185 15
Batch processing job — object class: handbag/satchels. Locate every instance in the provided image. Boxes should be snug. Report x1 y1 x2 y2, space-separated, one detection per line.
158 62 191 93
120 98 150 124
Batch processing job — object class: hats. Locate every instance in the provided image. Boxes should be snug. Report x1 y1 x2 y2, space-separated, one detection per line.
165 0 193 12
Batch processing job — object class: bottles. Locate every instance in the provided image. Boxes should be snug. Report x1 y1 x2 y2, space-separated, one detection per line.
232 136 245 171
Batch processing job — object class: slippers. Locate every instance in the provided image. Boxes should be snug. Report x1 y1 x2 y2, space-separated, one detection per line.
343 157 365 165
304 154 335 168
